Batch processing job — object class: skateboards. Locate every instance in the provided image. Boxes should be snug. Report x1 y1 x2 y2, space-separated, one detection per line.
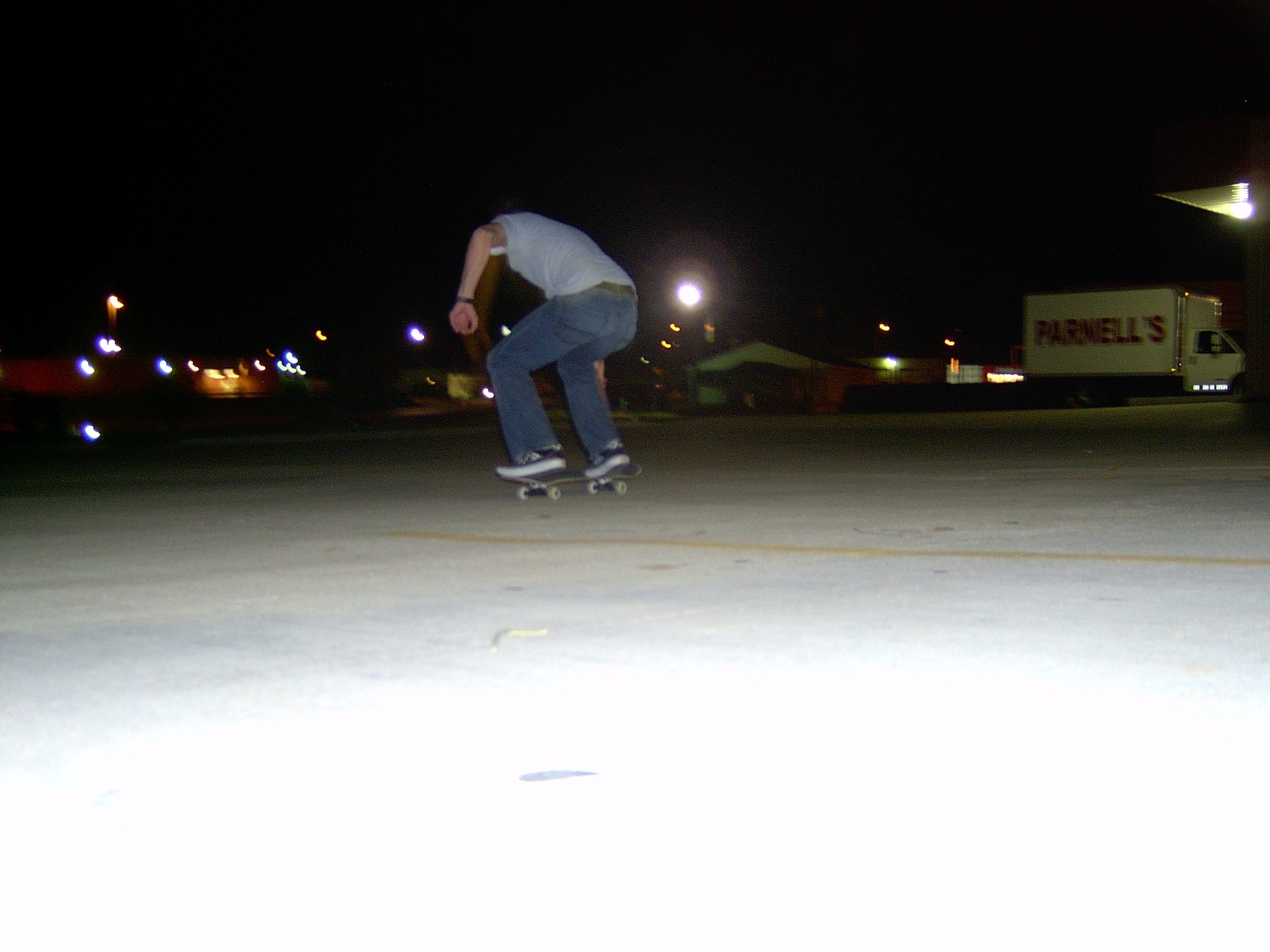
498 464 642 500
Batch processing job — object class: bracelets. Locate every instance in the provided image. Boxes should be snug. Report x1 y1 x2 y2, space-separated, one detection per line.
455 295 476 305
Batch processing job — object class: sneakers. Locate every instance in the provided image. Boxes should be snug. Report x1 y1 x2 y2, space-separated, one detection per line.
495 444 568 479
586 446 630 479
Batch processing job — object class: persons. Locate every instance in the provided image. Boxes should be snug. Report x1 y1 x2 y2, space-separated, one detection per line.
449 204 639 477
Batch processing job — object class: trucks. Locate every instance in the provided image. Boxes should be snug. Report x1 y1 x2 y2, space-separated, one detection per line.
1020 286 1252 403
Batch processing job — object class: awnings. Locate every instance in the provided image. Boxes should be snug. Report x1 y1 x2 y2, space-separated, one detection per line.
1153 184 1232 215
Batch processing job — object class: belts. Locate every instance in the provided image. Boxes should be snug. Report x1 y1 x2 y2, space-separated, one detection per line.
596 279 634 295
